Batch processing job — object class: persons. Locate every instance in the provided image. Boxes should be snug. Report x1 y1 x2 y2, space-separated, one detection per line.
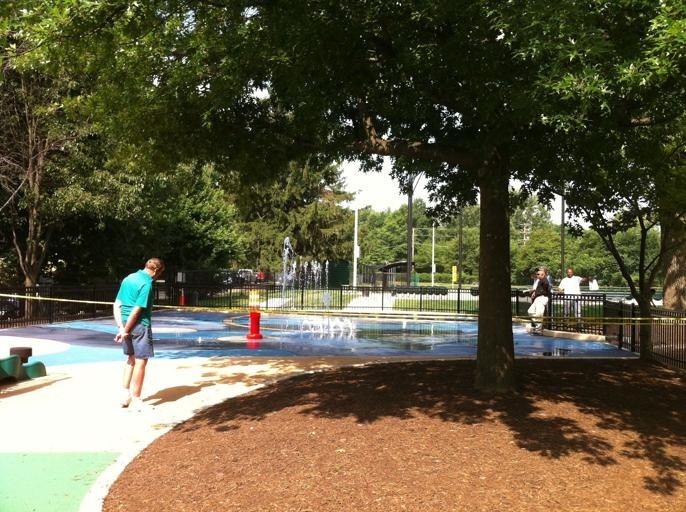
649 289 656 307
113 257 166 411
527 267 587 336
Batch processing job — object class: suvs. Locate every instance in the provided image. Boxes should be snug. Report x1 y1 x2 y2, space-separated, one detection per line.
236 268 254 283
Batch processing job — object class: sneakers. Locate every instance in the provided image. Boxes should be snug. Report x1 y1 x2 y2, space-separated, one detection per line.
531 327 543 334
128 399 155 412
118 392 132 406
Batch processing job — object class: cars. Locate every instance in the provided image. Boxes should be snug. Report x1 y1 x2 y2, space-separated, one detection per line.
218 269 244 288
0 299 20 320
255 271 268 281
